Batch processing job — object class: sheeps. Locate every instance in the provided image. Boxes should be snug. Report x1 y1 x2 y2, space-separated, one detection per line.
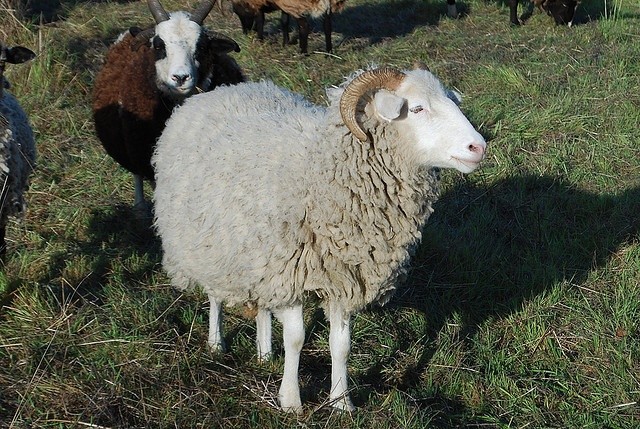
0 45 36 272
229 0 349 58
92 0 247 216
149 62 485 415
445 0 576 30
